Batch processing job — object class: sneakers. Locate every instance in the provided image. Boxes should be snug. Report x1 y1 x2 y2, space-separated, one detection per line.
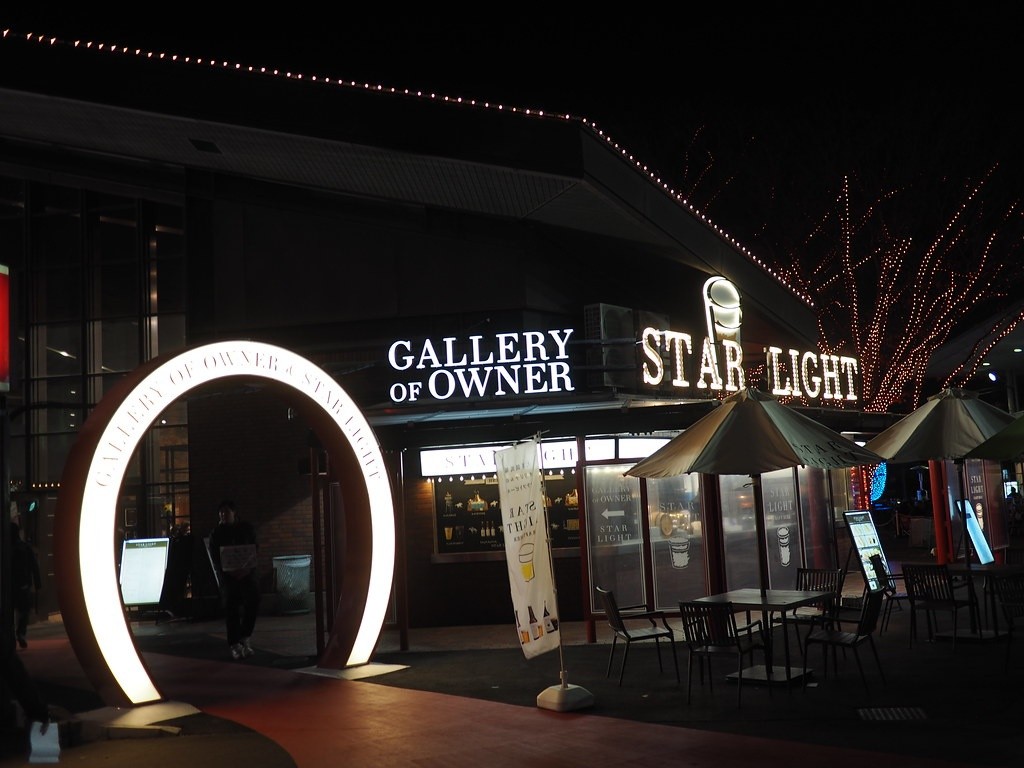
230 636 253 660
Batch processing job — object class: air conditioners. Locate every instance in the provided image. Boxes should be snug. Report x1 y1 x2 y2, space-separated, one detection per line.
635 310 671 340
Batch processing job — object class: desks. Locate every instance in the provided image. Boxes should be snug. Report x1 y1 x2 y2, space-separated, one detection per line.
695 589 841 695
949 564 1024 640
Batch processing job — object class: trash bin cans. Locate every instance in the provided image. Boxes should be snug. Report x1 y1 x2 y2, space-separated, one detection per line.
274 556 312 615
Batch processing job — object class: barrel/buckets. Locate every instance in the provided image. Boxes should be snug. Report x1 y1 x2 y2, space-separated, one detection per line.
648 512 673 537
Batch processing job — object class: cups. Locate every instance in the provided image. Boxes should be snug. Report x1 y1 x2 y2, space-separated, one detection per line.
522 630 529 643
445 528 452 539
538 625 544 637
551 618 559 630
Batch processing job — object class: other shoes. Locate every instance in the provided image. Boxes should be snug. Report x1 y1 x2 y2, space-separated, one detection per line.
16 630 27 648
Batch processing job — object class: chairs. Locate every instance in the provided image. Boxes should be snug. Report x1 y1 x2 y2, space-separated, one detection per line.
802 586 887 694
596 587 679 686
901 563 982 650
869 554 937 638
770 568 847 660
678 600 772 711
992 570 1024 673
984 547 1024 630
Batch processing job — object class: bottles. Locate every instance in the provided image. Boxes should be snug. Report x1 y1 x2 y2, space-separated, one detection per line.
515 610 525 644
527 606 539 640
445 492 453 514
491 521 495 536
486 521 490 537
468 489 488 512
543 600 555 634
481 521 485 537
468 497 473 504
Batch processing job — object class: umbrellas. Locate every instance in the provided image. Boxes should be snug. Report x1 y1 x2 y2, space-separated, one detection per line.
865 388 1006 634
623 381 870 672
961 412 1024 463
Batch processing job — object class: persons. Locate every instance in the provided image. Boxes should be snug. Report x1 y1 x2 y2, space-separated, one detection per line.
1009 487 1022 504
10 523 42 650
208 501 256 658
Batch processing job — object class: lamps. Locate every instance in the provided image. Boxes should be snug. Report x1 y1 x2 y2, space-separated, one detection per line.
584 303 634 340
586 344 636 389
